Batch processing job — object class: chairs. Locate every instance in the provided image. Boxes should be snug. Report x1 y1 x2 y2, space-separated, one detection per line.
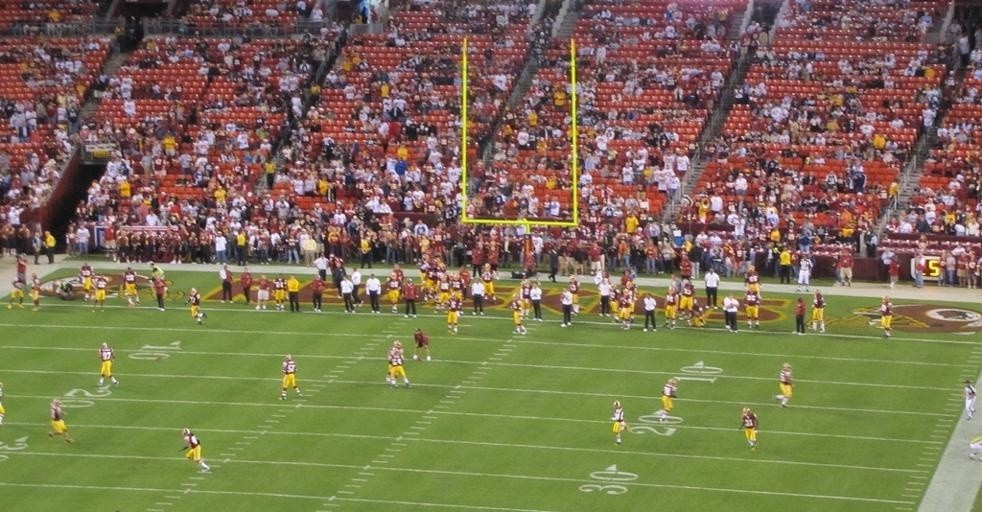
0 2 981 260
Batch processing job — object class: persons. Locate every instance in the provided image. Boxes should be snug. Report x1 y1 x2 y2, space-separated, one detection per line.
610 401 626 445
49 399 73 443
962 380 977 420
177 428 210 473
0 382 6 427
386 340 412 389
738 407 759 452
775 362 794 408
659 376 679 422
412 328 431 362
969 435 982 461
96 342 119 386
277 353 303 401
